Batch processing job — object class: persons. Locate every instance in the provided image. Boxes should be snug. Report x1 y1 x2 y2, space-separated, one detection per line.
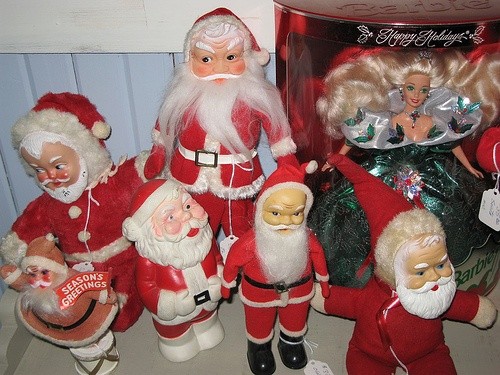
220 152 330 375
1 91 168 332
308 149 497 375
143 5 298 296
2 231 123 349
305 47 496 286
120 162 228 364
